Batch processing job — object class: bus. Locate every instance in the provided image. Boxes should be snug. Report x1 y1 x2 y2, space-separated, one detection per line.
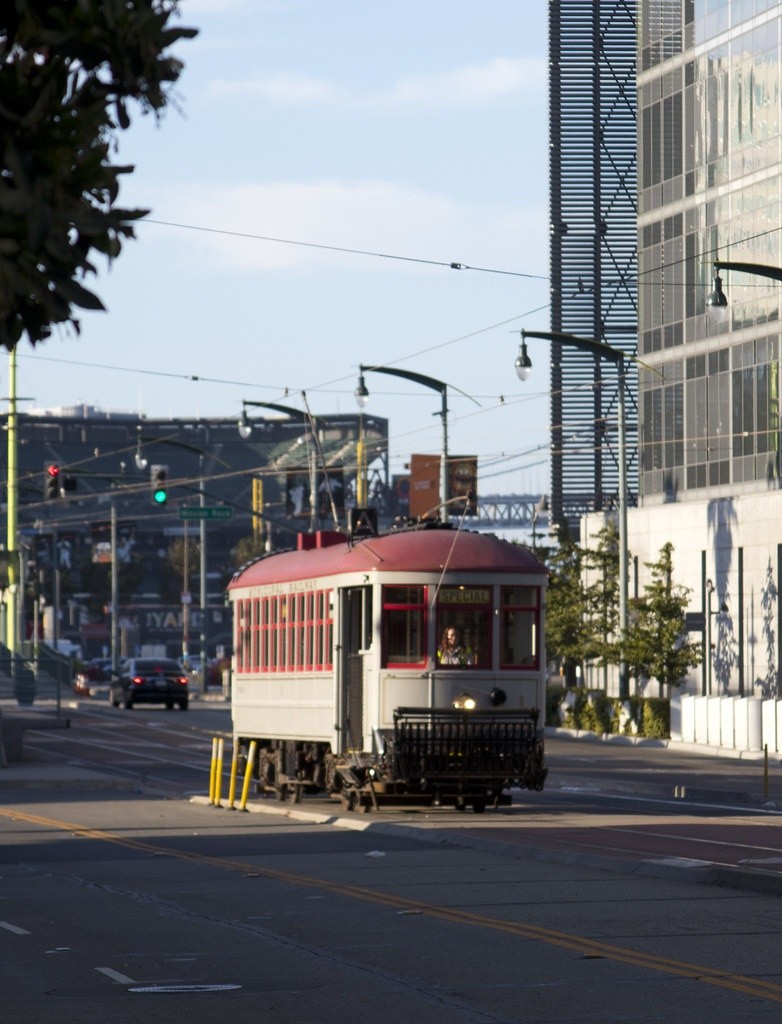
222 520 550 812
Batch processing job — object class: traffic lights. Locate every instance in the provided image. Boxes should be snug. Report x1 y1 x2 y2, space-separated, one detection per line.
43 460 61 500
150 464 169 506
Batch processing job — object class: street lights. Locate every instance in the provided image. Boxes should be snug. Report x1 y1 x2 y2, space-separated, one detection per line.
236 398 321 534
353 362 450 525
514 329 629 733
133 435 263 691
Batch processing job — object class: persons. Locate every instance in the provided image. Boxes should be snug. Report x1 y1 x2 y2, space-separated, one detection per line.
437 624 475 665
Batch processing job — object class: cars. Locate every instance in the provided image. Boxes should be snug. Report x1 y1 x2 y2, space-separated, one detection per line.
90 665 115 699
108 658 188 711
177 656 223 686
0 641 37 705
